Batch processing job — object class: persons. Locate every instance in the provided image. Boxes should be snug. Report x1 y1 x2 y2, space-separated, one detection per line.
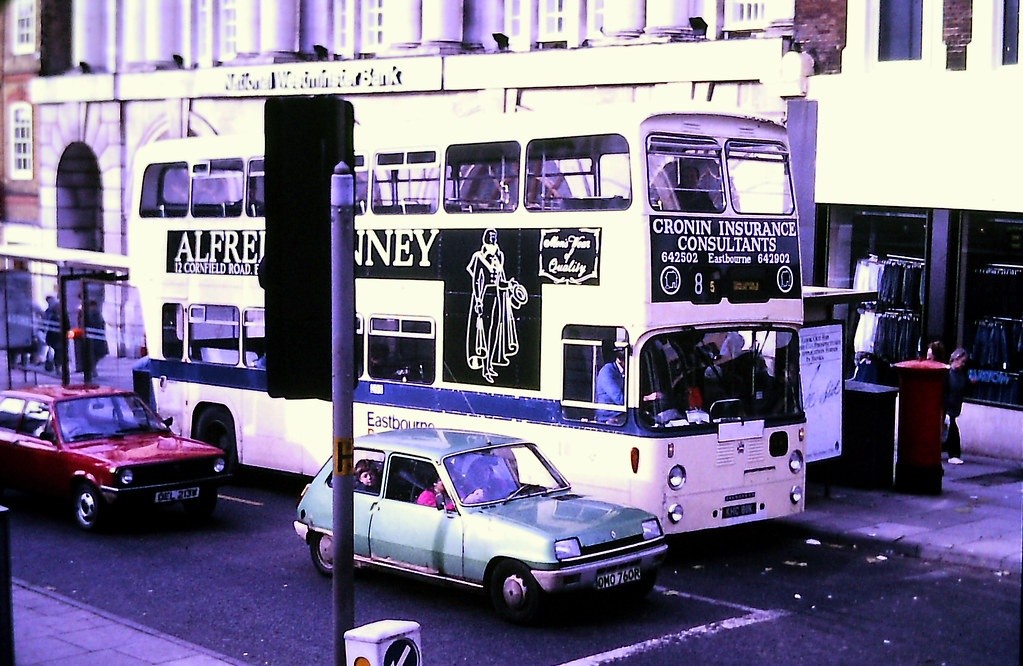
354 461 381 495
919 341 970 465
35 400 104 440
42 296 71 374
83 301 110 388
670 166 719 212
414 470 484 510
469 458 520 499
596 345 625 426
456 147 565 203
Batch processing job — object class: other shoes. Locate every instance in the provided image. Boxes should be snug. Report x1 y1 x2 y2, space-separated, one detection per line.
947 457 964 465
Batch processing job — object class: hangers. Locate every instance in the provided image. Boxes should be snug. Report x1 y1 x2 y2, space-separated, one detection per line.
868 255 921 268
880 308 918 321
980 316 1023 329
984 266 1023 275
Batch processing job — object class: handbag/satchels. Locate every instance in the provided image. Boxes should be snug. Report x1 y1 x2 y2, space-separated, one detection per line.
45 348 55 371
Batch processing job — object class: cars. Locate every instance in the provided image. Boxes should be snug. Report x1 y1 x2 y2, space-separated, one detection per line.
0 385 228 531
292 428 669 624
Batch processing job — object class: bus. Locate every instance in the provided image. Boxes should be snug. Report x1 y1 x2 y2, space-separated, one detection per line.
128 101 807 538
